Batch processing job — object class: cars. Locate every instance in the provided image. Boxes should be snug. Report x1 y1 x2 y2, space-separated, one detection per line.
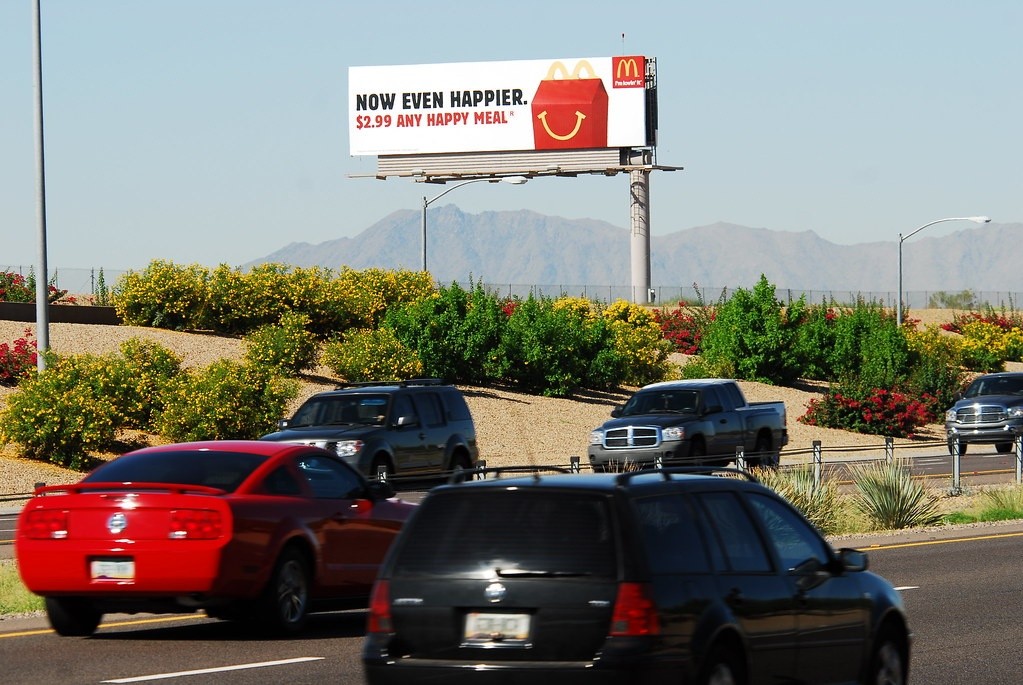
14 439 420 636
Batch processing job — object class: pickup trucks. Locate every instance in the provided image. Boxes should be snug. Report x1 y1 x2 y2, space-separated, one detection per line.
586 379 789 472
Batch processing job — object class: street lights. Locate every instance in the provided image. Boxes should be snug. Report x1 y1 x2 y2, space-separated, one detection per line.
896 216 992 328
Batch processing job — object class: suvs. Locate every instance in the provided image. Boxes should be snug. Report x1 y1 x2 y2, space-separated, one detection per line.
945 371 1023 455
360 465 912 684
261 378 480 496
